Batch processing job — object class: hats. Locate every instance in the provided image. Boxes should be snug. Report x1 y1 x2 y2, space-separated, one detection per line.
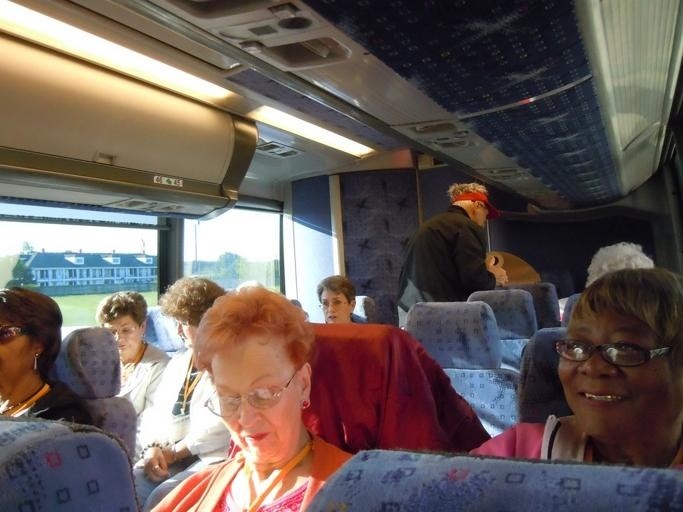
452 192 500 220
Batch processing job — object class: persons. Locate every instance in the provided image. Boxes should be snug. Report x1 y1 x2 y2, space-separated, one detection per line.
396 181 508 331
585 242 655 291
466 269 683 472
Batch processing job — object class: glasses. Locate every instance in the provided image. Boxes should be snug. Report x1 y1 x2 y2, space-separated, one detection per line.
319 301 348 310
553 340 673 367
0 325 28 343
206 370 298 416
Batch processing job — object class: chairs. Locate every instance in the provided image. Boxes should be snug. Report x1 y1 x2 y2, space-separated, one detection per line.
0 417 140 509
303 450 680 510
141 306 183 356
292 282 584 454
54 328 137 462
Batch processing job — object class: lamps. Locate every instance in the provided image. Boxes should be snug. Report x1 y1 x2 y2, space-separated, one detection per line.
0 2 387 167
